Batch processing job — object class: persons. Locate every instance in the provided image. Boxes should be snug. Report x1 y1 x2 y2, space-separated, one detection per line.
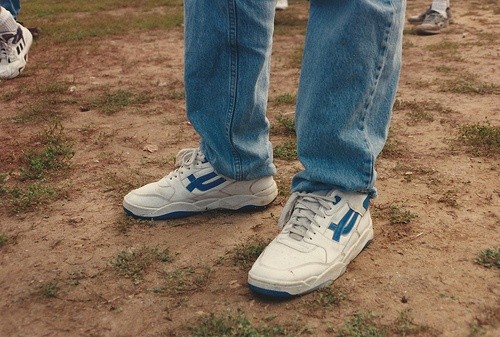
275 0 289 10
122 0 408 301
0 0 33 79
407 0 455 35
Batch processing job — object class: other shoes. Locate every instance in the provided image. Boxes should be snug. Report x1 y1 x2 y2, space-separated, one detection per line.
276 0 289 10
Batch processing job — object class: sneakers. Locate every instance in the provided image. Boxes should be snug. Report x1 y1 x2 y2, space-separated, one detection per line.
246 186 375 298
0 23 33 78
414 12 450 34
406 6 450 23
121 140 278 221
